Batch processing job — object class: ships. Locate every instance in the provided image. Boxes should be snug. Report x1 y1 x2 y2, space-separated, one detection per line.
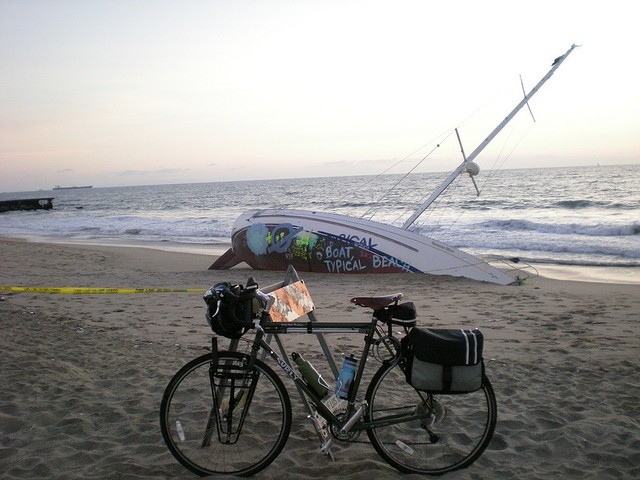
52 185 92 189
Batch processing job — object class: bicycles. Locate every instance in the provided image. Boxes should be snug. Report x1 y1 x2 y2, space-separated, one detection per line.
160 284 496 478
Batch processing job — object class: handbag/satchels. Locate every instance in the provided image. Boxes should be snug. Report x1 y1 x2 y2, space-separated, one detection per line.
402 328 485 393
204 283 244 339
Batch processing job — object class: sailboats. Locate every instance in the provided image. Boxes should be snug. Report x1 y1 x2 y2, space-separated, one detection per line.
207 44 581 285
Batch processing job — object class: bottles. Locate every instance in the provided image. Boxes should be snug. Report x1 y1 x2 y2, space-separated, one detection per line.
291 351 330 397
335 354 358 397
